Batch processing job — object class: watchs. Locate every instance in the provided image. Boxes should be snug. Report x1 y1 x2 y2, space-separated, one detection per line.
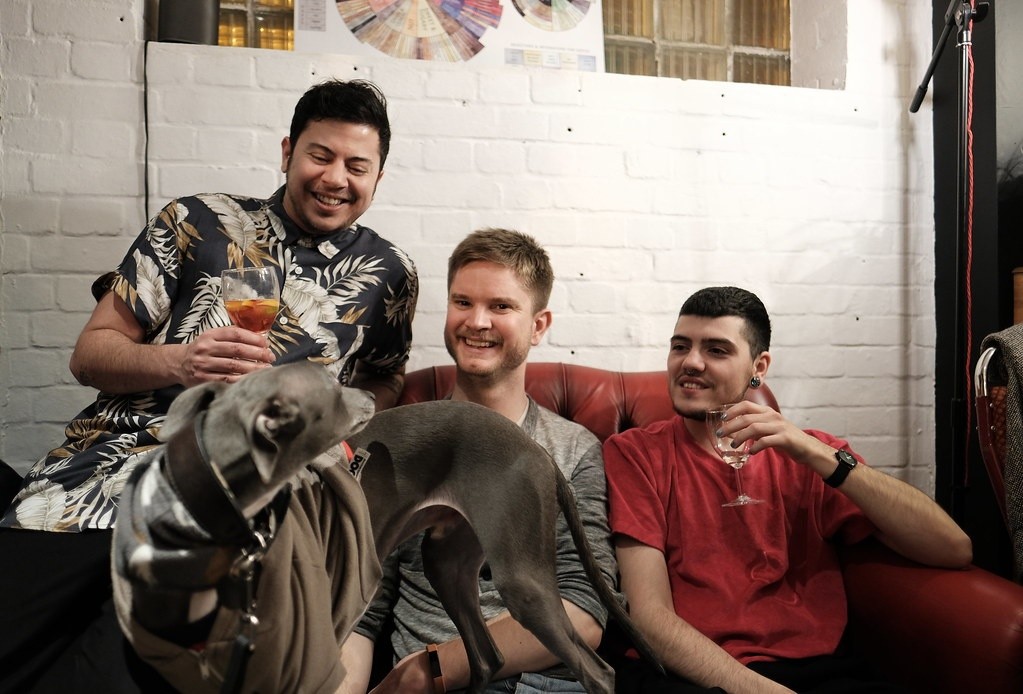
822 450 858 489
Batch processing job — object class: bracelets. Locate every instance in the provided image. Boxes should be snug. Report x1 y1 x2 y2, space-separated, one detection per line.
426 643 446 693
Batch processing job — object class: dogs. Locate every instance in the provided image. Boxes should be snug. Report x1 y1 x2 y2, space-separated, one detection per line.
110 360 667 694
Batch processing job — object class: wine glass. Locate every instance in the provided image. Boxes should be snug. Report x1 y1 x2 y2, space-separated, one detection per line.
221 267 280 368
706 404 766 508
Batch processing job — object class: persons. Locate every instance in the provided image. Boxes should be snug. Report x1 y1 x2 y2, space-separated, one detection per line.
0 78 419 694
604 287 975 694
331 230 621 693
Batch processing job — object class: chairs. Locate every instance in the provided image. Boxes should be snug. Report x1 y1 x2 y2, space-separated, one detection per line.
974 324 1023 572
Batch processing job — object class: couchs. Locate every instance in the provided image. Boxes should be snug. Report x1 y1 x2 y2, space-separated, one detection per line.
387 364 1022 693
